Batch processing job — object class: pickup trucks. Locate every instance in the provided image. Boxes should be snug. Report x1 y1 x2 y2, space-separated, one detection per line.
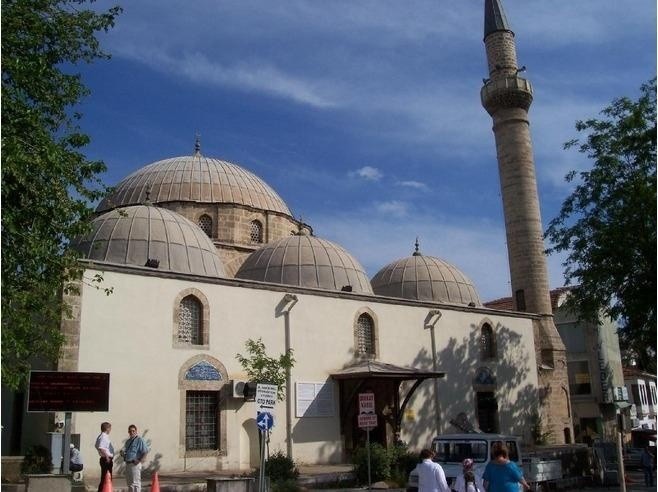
408 433 565 491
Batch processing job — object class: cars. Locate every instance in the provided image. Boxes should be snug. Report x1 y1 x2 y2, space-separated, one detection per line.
622 447 647 469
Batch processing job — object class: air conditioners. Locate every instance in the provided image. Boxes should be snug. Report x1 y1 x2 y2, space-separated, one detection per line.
232 380 255 400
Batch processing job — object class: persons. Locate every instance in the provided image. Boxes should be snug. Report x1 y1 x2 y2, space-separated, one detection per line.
639 445 655 488
455 458 485 492
446 477 456 492
458 472 482 492
482 441 531 492
415 450 452 492
94 422 115 492
69 443 83 471
119 425 149 492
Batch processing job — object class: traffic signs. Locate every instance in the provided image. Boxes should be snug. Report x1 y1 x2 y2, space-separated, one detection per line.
256 384 278 413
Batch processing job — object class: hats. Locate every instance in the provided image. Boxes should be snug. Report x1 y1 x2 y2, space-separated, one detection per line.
463 459 474 470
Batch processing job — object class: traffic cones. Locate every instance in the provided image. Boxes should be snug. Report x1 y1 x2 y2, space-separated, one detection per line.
149 471 160 491
101 470 113 492
